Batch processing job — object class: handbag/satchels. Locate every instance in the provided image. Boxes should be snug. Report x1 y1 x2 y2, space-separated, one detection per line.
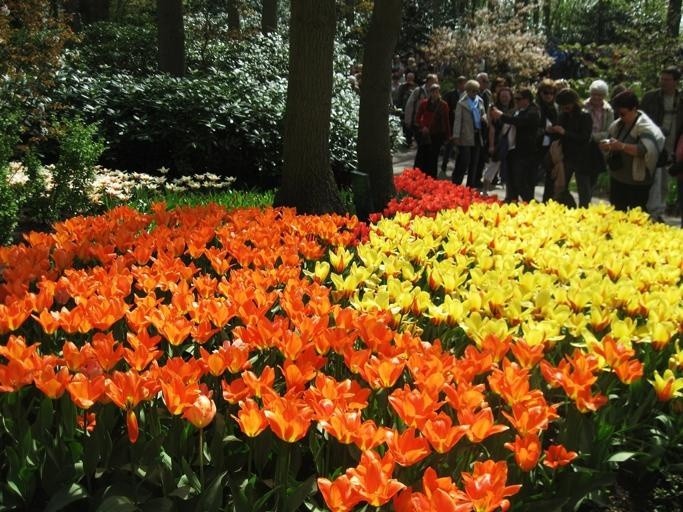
656 149 668 167
415 128 432 146
570 139 607 175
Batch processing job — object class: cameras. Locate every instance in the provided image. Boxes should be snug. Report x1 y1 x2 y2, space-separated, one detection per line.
489 103 496 110
600 139 613 145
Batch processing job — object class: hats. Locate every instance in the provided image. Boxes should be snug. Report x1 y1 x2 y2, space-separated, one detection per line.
514 89 532 99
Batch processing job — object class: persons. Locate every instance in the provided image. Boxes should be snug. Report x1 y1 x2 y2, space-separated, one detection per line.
641 66 682 224
348 55 433 148
405 72 662 208
